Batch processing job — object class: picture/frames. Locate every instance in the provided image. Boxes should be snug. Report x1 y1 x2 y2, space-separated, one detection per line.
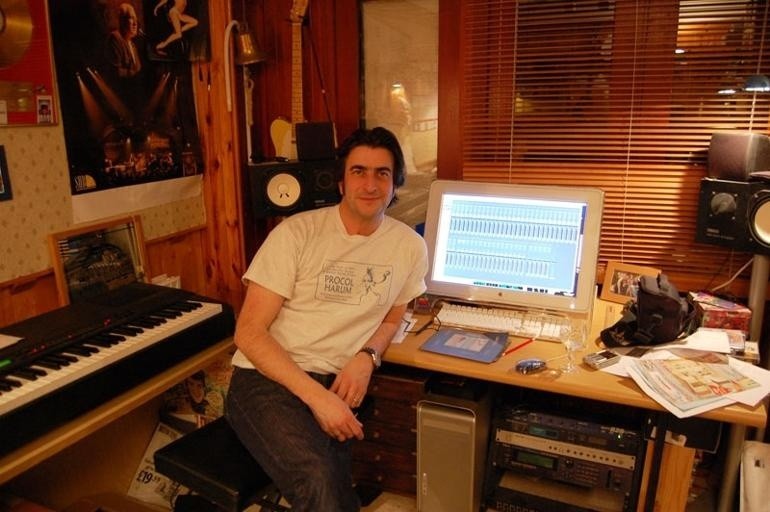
602 261 661 304
48 213 151 308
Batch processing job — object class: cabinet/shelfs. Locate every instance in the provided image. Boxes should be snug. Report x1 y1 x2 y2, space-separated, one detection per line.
352 375 427 500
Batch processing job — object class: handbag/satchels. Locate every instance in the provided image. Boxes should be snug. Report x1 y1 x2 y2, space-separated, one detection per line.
601 264 687 348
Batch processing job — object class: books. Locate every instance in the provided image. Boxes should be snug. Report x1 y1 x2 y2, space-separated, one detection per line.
695 326 761 366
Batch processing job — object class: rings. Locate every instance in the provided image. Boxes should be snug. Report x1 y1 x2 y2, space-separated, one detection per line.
354 398 360 404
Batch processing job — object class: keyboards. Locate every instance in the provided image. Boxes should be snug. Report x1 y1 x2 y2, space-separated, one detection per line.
433 301 566 343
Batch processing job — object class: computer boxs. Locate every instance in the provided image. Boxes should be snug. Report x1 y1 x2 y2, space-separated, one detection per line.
415 388 489 512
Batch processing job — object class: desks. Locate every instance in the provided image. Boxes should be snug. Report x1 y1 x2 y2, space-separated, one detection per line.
383 160 437 236
379 307 767 512
1 335 236 483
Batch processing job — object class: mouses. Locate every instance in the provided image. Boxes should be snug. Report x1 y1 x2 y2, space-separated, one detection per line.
519 359 544 373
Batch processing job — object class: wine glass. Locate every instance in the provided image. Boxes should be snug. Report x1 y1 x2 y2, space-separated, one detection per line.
559 317 588 377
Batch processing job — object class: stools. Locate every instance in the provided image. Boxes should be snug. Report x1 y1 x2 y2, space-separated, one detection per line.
153 415 292 512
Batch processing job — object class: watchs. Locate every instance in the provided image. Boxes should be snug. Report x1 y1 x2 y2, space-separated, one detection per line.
354 347 382 372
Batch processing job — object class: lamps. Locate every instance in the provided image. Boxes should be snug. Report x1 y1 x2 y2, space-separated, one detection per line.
745 74 770 91
390 84 417 172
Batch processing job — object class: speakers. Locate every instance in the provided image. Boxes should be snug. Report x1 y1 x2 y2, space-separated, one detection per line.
247 160 341 220
708 131 769 177
295 121 332 159
695 177 770 257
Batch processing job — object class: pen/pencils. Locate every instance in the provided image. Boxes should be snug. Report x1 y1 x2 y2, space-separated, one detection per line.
250 151 291 163
415 320 433 337
502 338 535 357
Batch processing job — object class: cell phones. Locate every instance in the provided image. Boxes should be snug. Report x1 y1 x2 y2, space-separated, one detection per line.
582 350 620 370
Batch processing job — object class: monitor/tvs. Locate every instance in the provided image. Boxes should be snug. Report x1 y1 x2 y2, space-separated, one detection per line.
421 180 605 315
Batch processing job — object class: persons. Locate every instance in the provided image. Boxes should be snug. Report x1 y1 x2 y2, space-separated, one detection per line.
614 273 640 297
102 3 144 84
151 1 199 56
226 126 431 510
